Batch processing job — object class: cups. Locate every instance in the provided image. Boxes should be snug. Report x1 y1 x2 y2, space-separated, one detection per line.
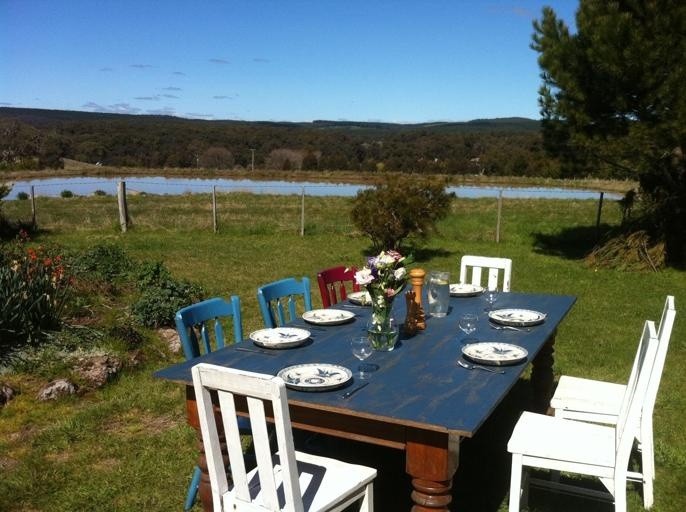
428 271 450 318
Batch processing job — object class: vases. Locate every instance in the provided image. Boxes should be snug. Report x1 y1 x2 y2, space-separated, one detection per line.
365 295 400 352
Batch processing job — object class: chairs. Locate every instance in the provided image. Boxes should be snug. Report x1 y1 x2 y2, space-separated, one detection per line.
459 255 512 293
258 277 312 329
549 294 677 512
317 266 358 309
192 361 378 512
174 295 250 512
506 319 660 512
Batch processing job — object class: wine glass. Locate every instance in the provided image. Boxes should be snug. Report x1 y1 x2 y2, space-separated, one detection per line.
351 338 373 380
459 315 479 343
484 291 498 313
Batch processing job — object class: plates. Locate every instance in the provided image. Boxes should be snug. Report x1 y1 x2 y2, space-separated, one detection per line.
277 363 353 391
302 308 356 326
488 308 546 327
348 291 375 304
461 342 526 366
450 284 485 296
250 327 312 349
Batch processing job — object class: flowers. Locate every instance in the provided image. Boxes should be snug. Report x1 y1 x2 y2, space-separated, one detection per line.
354 249 412 333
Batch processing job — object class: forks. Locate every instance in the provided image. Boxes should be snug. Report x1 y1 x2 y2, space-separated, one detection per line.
458 361 506 374
489 322 531 332
